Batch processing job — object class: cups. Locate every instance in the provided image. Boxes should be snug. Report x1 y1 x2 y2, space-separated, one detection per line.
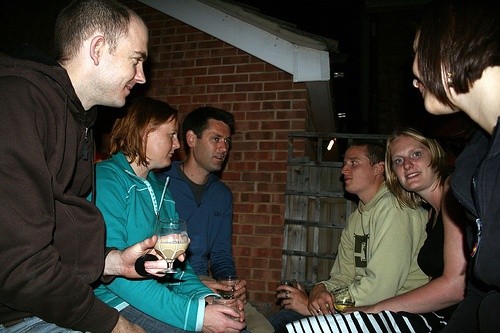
212 296 240 322
330 285 355 313
218 277 239 295
279 279 297 294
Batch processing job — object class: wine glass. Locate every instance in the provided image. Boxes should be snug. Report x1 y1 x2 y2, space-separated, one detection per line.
152 219 188 274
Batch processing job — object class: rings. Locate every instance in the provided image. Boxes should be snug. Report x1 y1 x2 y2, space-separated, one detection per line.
316 307 322 313
285 291 289 298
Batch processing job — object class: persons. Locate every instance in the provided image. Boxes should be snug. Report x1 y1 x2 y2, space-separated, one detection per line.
275 0 500 333
86 98 246 333
272 138 429 333
342 128 478 314
0 0 186 332
153 106 276 333
